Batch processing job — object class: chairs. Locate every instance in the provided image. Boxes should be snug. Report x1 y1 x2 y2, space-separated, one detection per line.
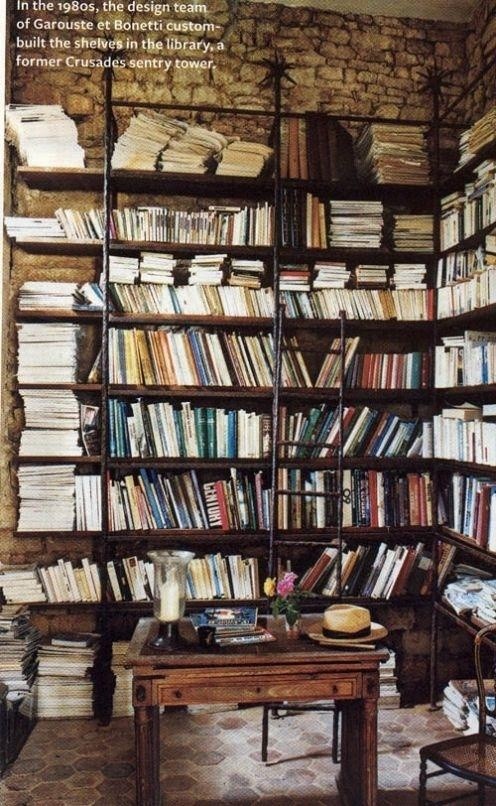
419 622 494 806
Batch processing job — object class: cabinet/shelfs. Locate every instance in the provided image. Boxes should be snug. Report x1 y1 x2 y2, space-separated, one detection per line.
2 101 494 776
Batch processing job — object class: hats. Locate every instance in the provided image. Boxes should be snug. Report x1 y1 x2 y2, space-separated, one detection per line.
303 603 388 643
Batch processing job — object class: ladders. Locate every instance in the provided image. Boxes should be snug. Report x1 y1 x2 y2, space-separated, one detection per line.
268 303 345 611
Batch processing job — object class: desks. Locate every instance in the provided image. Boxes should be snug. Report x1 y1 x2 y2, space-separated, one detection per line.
123 613 390 805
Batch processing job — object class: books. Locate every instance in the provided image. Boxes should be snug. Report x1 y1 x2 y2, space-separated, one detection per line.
0 103 495 735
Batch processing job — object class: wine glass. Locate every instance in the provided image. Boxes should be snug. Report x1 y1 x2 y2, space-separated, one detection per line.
145 548 196 652
